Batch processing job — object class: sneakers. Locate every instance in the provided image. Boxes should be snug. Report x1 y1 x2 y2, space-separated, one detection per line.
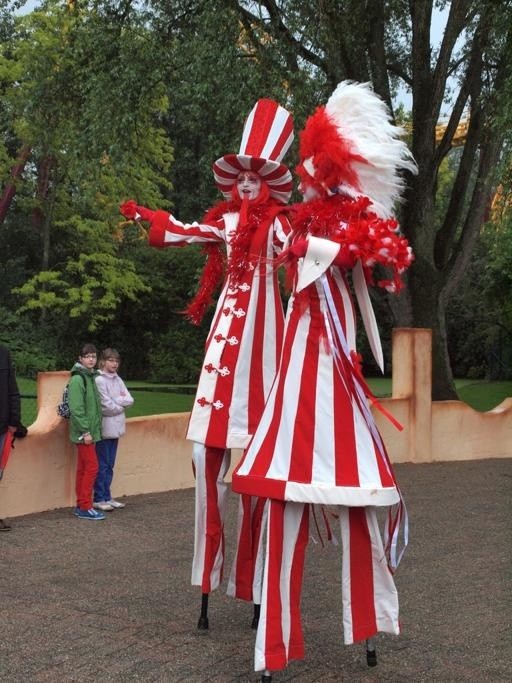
93 501 113 510
0 519 11 531
78 508 105 520
106 499 125 508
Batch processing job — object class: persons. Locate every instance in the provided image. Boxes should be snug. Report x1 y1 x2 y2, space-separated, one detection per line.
118 151 297 590
67 343 106 520
95 347 135 511
0 341 22 532
233 88 400 641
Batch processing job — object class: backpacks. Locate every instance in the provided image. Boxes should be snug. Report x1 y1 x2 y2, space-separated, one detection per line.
57 370 86 418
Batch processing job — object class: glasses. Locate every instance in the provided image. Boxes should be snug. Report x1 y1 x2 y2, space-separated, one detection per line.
83 354 97 360
